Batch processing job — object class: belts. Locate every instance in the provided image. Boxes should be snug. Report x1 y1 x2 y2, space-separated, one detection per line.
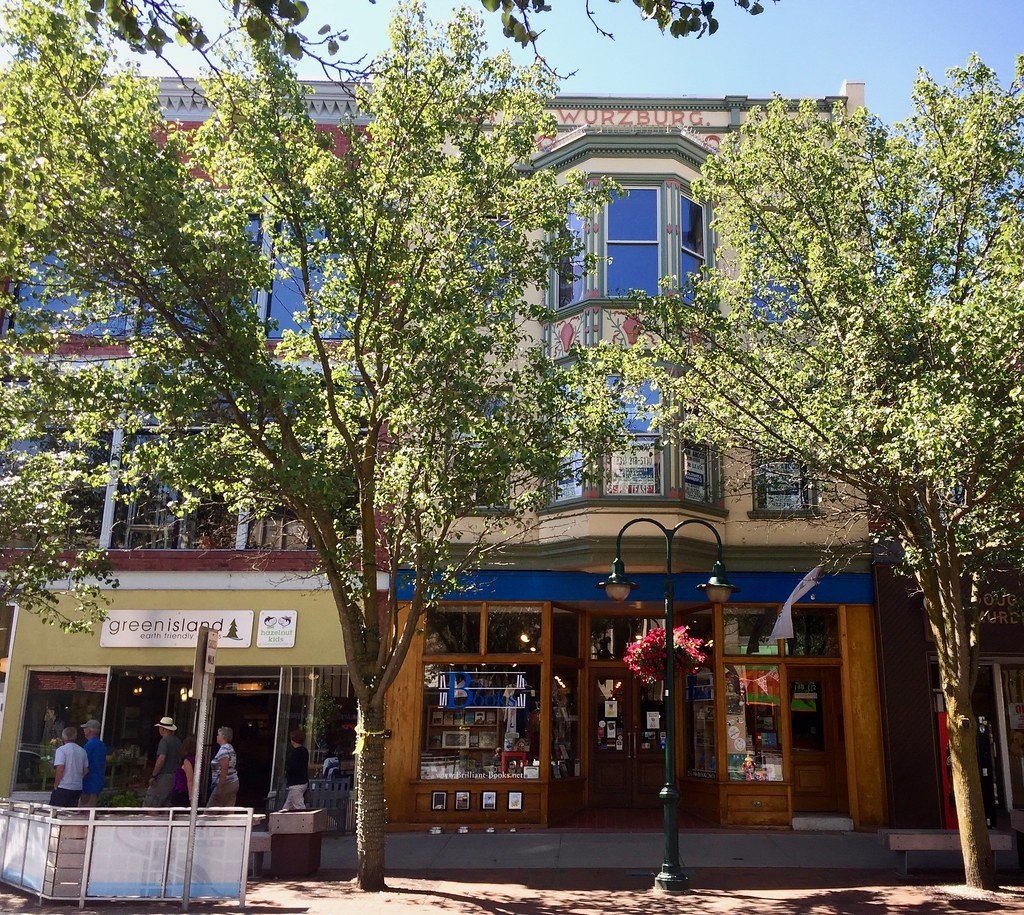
159 771 178 773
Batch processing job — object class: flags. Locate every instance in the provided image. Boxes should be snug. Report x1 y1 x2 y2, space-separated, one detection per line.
769 562 819 641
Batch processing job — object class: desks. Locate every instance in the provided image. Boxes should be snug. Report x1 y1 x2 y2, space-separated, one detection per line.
105 755 147 788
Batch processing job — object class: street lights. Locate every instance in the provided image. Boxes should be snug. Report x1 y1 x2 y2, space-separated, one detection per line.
598 518 740 895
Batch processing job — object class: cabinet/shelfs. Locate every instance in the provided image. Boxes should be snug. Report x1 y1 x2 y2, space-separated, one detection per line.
693 699 715 773
425 705 505 750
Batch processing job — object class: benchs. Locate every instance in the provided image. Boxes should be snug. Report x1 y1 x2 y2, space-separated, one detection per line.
876 829 1012 880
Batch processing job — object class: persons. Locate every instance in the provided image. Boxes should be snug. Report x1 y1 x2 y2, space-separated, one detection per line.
47 726 89 808
203 727 239 815
172 736 205 814
79 719 107 807
321 747 345 790
140 716 183 814
282 730 310 810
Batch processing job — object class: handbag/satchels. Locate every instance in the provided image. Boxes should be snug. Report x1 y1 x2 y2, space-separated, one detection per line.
210 783 215 790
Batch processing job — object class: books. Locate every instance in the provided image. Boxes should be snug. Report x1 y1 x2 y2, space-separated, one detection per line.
454 712 464 725
465 712 474 724
444 713 453 725
432 711 443 725
486 712 495 724
475 712 485 724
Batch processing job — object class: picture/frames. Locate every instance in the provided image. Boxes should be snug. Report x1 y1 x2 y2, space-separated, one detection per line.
455 790 471 811
474 711 486 725
507 790 524 811
481 790 497 811
441 730 470 749
431 790 448 810
477 731 496 749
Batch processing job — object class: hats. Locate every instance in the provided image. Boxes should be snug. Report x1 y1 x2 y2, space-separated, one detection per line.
80 720 100 729
154 716 177 731
